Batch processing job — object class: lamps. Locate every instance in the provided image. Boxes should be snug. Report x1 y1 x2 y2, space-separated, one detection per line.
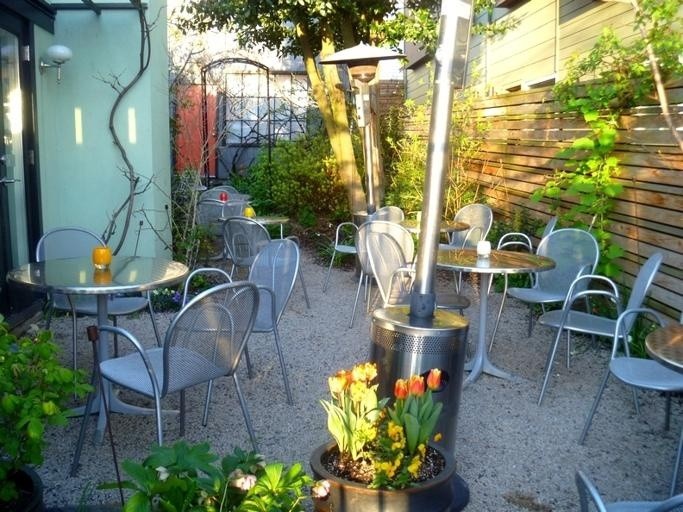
36 44 74 82
318 43 407 286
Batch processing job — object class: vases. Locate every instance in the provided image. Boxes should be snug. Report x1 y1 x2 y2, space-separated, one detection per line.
310 438 458 507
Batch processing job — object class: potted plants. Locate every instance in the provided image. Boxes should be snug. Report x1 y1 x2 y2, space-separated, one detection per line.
0 320 94 512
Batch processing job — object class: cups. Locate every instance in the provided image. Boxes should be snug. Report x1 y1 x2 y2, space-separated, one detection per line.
476 239 492 255
243 205 255 220
475 256 490 268
93 268 112 286
220 191 227 201
93 247 112 271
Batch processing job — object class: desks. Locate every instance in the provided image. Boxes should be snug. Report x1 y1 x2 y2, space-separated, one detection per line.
434 248 556 392
247 215 289 237
643 323 680 373
395 217 472 243
9 254 193 452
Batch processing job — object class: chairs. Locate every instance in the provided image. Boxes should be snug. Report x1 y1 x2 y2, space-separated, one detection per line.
363 229 471 357
579 308 683 447
347 220 416 327
487 216 559 294
537 250 663 445
448 203 492 296
321 206 406 301
35 228 168 403
488 228 601 406
72 280 261 477
170 238 301 425
223 217 310 314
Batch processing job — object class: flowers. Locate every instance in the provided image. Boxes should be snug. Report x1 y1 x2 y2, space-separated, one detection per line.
319 360 448 488
94 443 330 512
